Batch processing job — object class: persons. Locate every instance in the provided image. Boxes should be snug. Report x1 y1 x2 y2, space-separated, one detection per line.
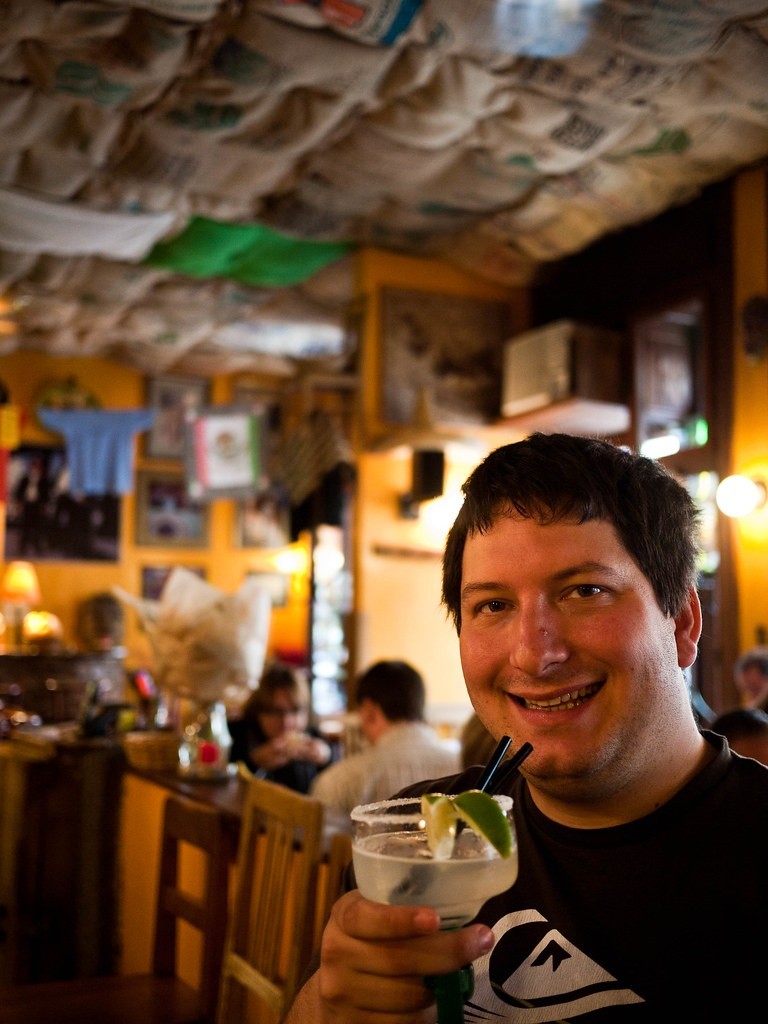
281 429 768 1024
6 461 117 565
0 593 466 833
703 649 768 753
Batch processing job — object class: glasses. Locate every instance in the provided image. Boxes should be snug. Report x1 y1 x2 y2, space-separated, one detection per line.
260 705 308 718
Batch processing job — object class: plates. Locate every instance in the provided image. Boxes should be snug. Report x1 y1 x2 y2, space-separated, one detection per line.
172 762 241 782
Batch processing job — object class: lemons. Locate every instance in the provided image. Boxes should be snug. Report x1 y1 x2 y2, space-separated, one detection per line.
420 792 458 860
450 790 512 860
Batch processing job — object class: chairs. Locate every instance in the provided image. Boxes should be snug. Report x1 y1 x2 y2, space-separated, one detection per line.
2 776 355 1024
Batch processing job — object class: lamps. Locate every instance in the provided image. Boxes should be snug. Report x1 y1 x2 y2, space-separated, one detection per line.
2 559 40 650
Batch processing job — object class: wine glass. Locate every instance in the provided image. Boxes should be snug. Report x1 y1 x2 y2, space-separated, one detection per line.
350 792 519 1024
